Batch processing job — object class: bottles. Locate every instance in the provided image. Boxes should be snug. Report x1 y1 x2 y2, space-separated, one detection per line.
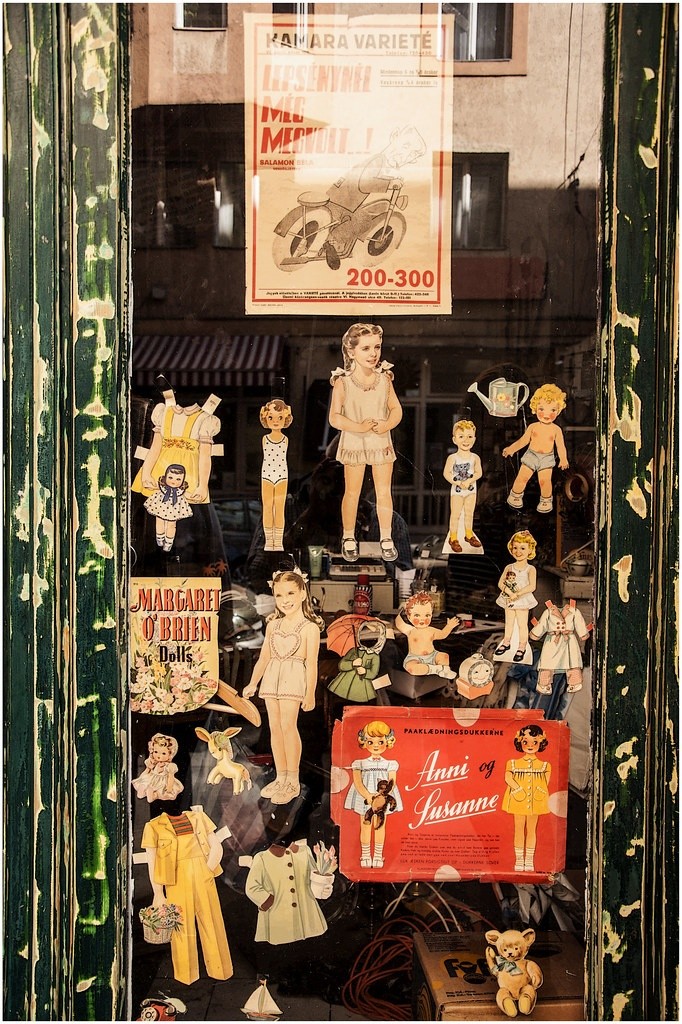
354 573 372 617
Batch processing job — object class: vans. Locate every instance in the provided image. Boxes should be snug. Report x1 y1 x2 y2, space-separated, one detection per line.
134 493 411 644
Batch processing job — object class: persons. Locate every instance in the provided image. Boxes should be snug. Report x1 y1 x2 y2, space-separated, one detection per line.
130 431 414 820
